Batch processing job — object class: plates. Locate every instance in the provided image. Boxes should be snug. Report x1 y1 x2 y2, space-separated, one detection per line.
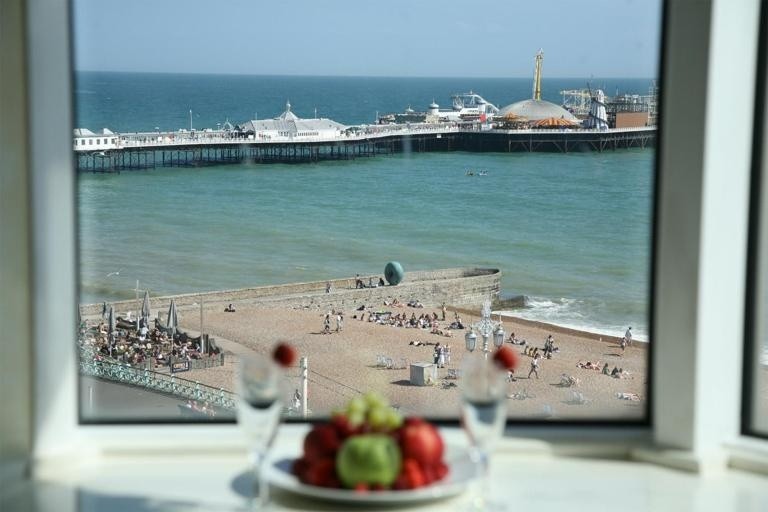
263 445 476 504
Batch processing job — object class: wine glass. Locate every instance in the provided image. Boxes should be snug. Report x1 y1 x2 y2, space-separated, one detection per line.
231 354 287 512
455 357 512 480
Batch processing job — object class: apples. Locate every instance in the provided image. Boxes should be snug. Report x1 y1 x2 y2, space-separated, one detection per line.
334 434 401 486
398 421 444 468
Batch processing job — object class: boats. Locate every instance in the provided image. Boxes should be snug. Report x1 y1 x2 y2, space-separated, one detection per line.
374 90 499 123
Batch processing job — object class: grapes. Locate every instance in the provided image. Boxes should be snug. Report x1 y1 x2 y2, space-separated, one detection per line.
331 392 404 431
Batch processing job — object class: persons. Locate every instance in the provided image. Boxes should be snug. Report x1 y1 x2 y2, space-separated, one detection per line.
324 314 332 335
185 400 215 417
335 312 343 333
78 318 218 367
356 298 641 406
378 278 384 286
294 389 301 399
325 281 333 294
356 274 366 288
228 303 236 312
368 277 374 288
